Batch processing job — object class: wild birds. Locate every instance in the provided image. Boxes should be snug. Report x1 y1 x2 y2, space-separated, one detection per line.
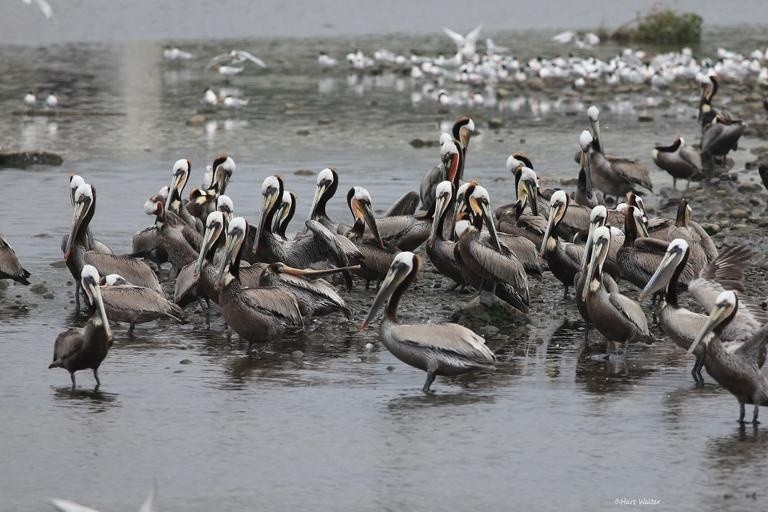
0 233 33 288
215 63 245 81
162 42 192 65
201 86 217 106
708 72 737 122
45 88 60 107
19 0 58 19
223 93 251 108
696 80 713 121
23 89 36 108
312 27 766 121
206 48 270 69
47 103 768 427
